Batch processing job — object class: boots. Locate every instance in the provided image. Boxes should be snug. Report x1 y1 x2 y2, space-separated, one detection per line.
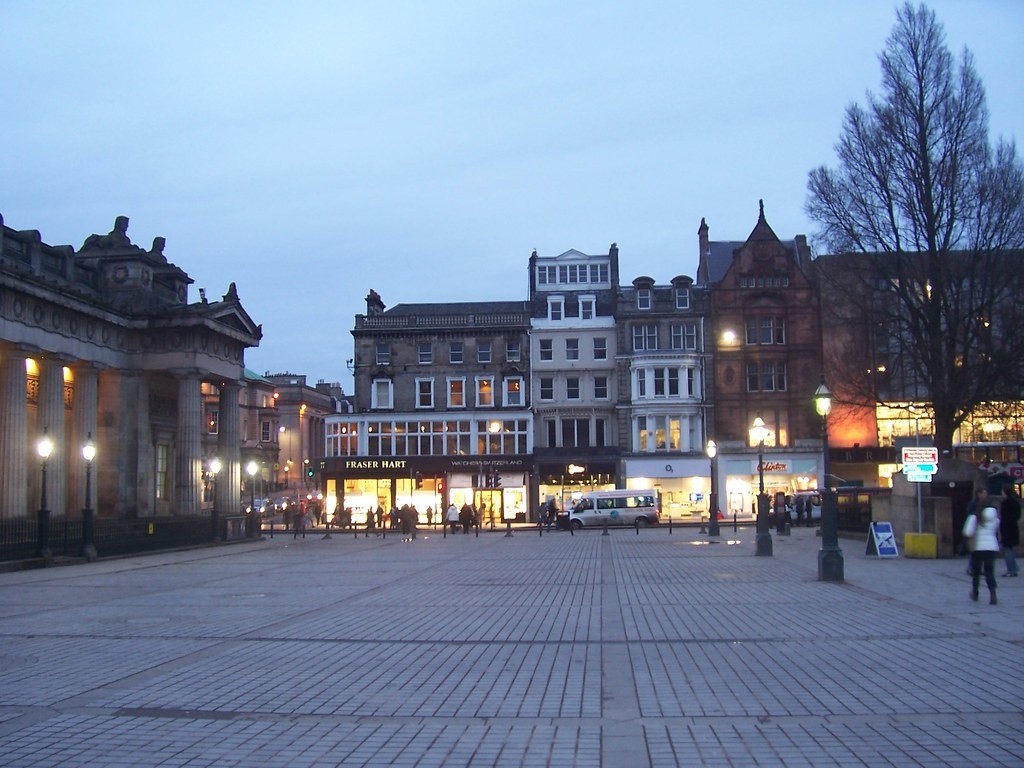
990 591 997 604
970 591 978 601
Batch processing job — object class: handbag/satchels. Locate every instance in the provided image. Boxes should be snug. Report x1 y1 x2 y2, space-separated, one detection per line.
961 513 977 538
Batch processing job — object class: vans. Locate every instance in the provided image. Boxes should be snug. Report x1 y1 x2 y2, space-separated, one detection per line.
563 489 658 529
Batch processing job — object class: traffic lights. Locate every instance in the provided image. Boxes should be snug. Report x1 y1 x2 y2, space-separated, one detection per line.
486 473 492 487
495 470 501 487
416 471 423 489
309 468 313 477
438 483 444 492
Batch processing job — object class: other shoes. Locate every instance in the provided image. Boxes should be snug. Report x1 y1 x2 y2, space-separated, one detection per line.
1002 570 1017 577
967 568 974 577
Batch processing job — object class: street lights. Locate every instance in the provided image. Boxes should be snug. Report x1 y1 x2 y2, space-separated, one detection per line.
753 411 773 557
211 457 221 541
82 431 96 555
37 425 52 556
812 374 844 582
247 461 258 536
707 440 720 536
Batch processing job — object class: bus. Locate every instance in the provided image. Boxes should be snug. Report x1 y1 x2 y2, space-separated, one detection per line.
784 486 893 522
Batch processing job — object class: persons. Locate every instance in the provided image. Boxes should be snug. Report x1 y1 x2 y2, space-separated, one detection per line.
962 500 1000 605
965 482 993 575
994 483 1020 576
761 494 812 528
241 499 561 536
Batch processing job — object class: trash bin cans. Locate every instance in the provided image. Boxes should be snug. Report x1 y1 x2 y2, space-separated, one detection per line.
246 512 262 536
556 511 571 529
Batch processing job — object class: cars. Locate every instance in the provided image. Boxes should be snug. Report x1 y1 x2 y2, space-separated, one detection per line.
246 490 321 518
